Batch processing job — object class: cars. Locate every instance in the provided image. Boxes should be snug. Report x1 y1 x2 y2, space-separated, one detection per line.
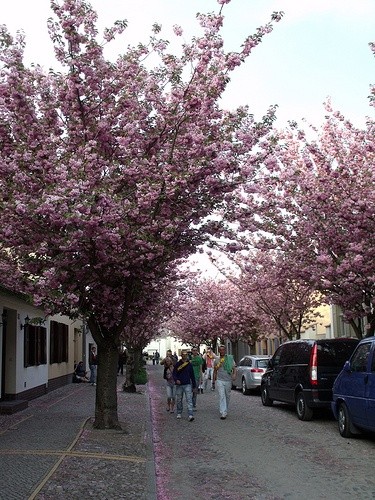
232 354 273 395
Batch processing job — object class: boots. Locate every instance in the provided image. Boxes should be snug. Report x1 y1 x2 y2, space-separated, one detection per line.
197 388 203 394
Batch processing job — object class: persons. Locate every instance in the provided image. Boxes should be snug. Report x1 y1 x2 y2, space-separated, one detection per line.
152 352 156 365
161 350 176 367
117 351 124 376
212 345 236 420
163 357 178 413
203 349 206 355
190 347 203 407
172 350 197 421
173 352 178 361
197 353 207 394
202 346 217 391
155 350 160 364
74 362 90 383
89 347 97 386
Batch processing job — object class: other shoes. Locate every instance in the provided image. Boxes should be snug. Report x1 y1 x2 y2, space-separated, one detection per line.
89 382 96 386
166 407 174 412
176 413 181 418
220 411 227 419
189 415 194 421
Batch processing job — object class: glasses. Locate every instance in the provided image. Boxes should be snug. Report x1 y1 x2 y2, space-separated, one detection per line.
182 353 187 355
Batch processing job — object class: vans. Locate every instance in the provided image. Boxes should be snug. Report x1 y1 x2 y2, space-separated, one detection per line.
261 336 363 420
329 337 375 438
